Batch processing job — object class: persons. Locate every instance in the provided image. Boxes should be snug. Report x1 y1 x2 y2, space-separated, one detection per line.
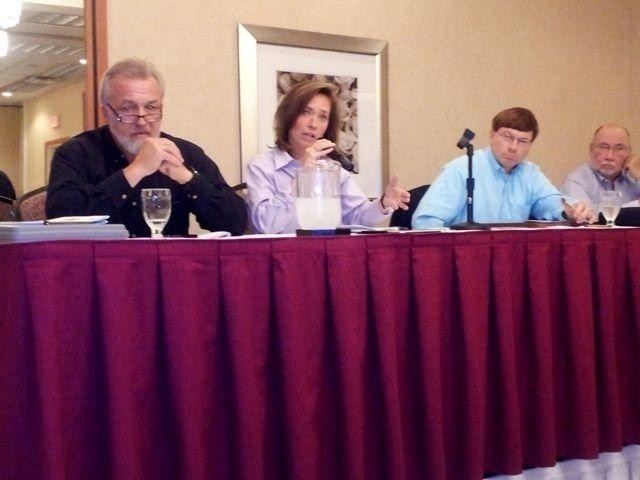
562 124 639 223
45 57 247 237
243 81 411 235
411 107 599 230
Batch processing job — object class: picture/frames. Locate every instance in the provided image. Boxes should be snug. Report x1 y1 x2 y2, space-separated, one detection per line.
235 18 392 205
43 136 70 186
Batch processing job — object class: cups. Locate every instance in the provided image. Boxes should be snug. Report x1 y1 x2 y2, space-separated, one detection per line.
296 165 342 231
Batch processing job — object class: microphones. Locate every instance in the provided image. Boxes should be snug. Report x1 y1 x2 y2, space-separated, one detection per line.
324 148 354 172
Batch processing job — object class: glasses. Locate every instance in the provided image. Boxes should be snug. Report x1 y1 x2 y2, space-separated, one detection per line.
106 102 163 124
594 141 630 153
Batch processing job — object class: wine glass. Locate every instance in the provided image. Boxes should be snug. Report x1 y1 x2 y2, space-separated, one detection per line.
598 190 623 228
142 188 172 237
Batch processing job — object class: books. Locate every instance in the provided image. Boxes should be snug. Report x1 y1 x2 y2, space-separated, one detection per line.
196 231 296 240
451 221 591 231
0 214 131 241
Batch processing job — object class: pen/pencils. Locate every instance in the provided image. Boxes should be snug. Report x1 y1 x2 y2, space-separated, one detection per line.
561 198 591 221
166 235 197 237
361 231 391 233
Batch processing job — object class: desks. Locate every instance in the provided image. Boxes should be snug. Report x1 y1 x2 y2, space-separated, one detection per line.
2 212 639 480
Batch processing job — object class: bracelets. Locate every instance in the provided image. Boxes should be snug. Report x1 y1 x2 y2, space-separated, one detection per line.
189 165 200 185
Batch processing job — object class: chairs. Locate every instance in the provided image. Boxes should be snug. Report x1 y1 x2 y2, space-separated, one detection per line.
13 180 50 224
389 181 432 229
